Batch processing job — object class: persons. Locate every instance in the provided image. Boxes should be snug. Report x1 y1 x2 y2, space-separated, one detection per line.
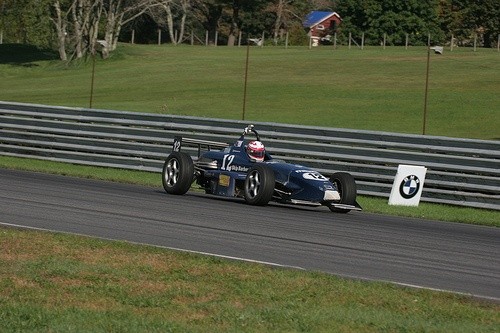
247 139 266 161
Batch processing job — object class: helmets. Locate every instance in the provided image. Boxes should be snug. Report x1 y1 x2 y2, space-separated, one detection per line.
247 140 265 163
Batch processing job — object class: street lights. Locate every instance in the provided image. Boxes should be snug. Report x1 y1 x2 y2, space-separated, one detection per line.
242 35 263 121
89 39 108 108
423 43 443 137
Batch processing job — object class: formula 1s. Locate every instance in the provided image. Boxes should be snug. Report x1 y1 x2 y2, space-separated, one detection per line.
162 120 364 215
